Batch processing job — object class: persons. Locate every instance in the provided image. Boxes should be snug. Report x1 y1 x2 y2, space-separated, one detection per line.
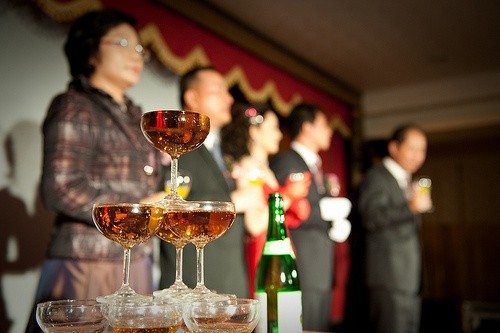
230 102 312 301
160 66 264 333
357 122 433 333
273 103 351 333
24 9 166 333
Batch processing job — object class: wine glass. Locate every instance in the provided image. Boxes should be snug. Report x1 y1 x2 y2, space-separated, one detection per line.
141 110 210 209
93 201 165 304
162 202 236 305
144 201 200 299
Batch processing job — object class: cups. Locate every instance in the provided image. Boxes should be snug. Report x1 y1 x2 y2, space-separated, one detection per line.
183 298 260 333
37 299 113 333
162 169 192 200
98 300 183 333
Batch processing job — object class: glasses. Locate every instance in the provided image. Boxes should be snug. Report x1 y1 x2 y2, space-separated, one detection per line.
100 38 145 56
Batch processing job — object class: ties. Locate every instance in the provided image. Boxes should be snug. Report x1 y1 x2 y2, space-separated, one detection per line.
314 161 325 195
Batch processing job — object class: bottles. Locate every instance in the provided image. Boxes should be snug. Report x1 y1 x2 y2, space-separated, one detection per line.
254 192 304 333
